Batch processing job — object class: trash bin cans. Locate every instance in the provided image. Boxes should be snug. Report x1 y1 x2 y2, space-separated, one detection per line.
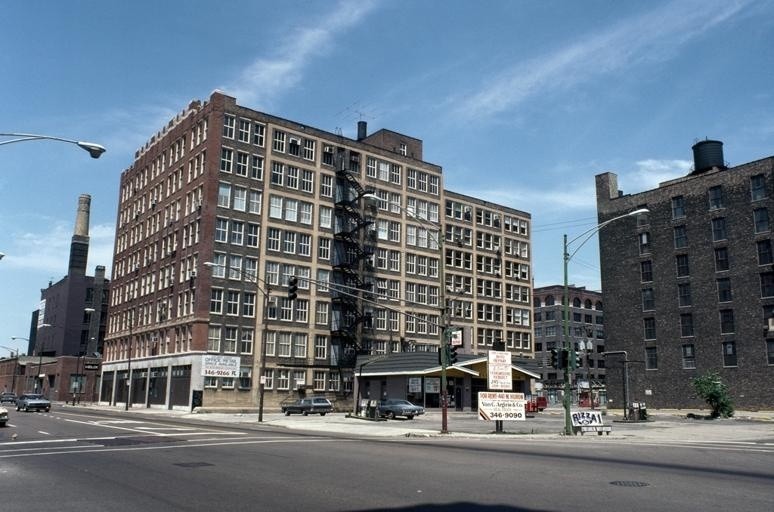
629 401 646 421
192 390 203 410
360 399 380 417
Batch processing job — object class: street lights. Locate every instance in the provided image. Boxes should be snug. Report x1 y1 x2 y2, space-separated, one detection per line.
563 208 652 436
203 262 270 422
85 307 132 411
38 322 83 406
11 336 43 393
0 131 107 159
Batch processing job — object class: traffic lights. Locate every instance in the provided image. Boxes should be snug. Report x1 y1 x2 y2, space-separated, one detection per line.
575 350 581 368
288 277 298 302
448 345 457 365
551 349 558 370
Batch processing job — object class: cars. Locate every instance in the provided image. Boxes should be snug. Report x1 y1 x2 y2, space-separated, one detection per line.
282 397 334 417
0 393 51 424
379 399 424 419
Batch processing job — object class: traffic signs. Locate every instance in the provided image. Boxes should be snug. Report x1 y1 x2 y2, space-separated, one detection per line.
362 193 448 436
